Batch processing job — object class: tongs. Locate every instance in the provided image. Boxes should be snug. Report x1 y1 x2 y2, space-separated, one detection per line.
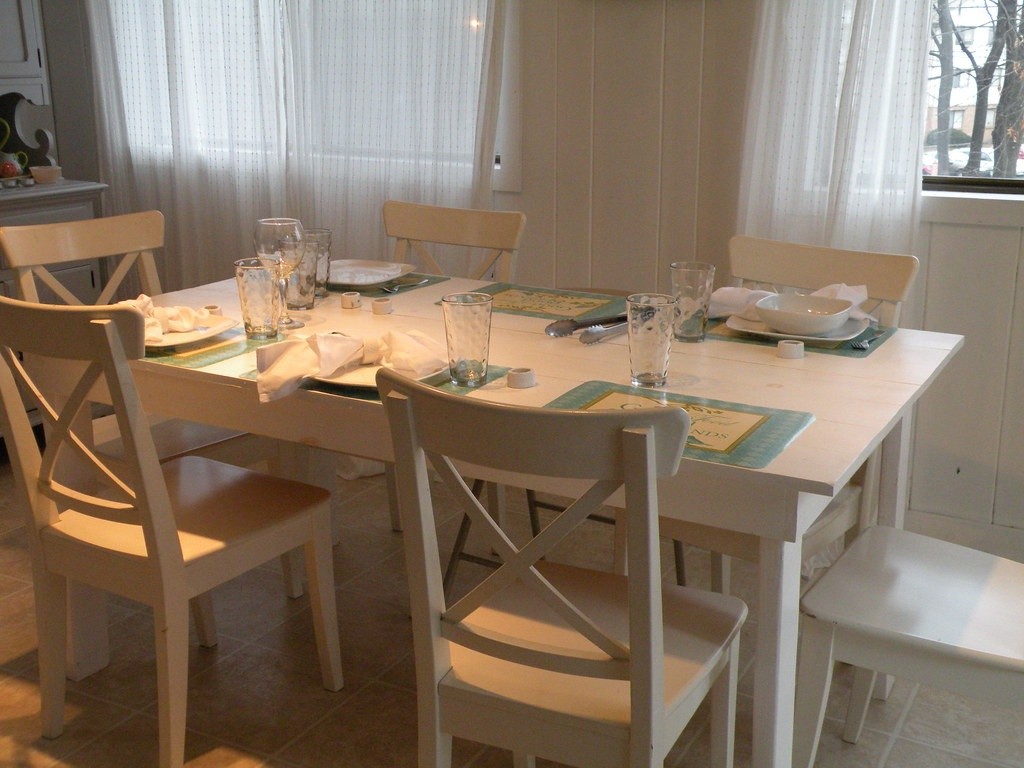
544 305 656 346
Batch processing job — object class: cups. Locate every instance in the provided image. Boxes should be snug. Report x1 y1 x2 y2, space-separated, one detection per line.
232 256 281 341
277 237 319 311
341 292 361 309
303 227 332 300
441 292 494 389
625 292 675 390
669 261 716 344
371 297 392 315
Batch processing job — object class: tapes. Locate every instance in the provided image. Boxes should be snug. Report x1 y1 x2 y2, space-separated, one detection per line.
507 368 534 388
202 305 223 315
778 341 805 360
341 291 361 309
372 298 392 316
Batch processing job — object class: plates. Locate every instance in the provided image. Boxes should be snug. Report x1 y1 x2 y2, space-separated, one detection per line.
90 313 240 352
726 315 870 349
309 356 460 387
298 259 417 292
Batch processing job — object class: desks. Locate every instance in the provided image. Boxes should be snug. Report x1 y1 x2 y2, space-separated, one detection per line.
19 273 966 768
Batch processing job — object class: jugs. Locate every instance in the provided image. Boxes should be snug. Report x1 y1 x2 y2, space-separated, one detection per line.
0 152 28 180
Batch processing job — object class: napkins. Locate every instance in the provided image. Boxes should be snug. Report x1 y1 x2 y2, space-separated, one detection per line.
112 294 210 344
709 281 878 325
253 328 449 404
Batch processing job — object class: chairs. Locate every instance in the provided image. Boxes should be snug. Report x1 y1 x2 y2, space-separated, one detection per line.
0 210 305 651
611 231 921 595
374 363 749 768
304 200 528 558
0 295 345 768
792 524 1024 768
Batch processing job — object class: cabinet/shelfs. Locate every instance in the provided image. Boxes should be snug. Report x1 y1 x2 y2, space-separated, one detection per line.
0 0 111 428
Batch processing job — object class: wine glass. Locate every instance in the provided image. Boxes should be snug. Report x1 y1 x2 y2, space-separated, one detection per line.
254 218 305 331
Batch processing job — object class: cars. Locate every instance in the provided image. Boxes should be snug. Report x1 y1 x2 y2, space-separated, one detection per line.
923 144 1024 178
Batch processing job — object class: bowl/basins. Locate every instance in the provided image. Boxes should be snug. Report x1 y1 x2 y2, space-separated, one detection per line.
29 165 61 183
755 294 854 336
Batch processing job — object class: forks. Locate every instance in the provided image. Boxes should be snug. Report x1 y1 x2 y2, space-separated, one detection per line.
378 279 429 293
850 330 887 351
